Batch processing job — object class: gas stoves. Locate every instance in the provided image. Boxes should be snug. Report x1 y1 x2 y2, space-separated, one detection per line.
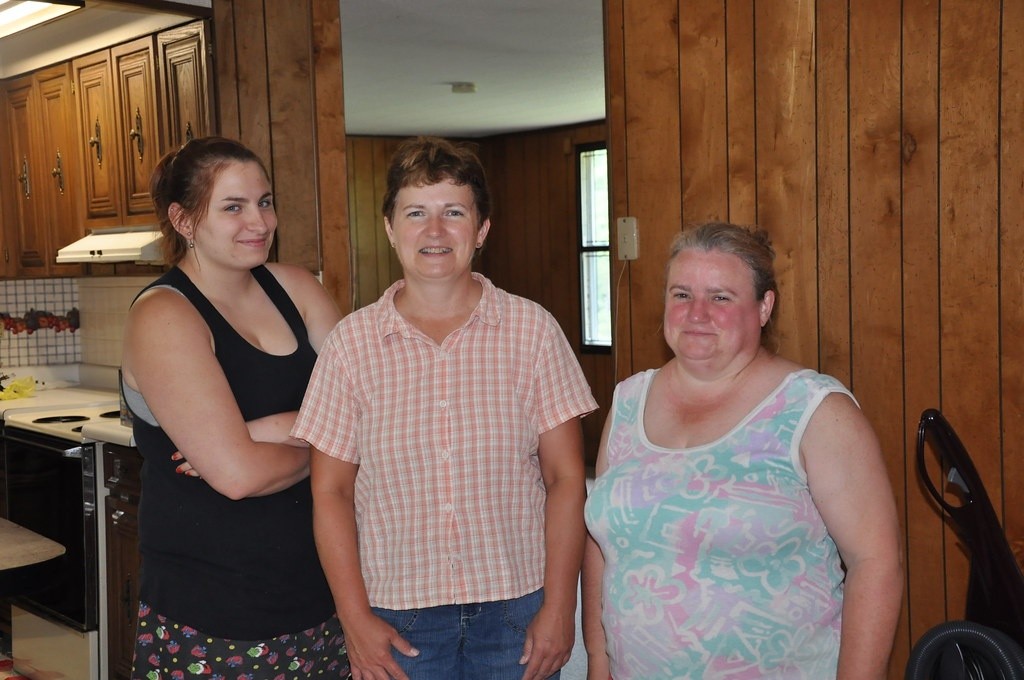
2 397 136 445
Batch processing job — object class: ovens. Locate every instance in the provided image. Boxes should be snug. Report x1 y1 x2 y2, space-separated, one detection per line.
0 423 97 632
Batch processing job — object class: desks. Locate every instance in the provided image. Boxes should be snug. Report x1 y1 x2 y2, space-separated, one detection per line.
0 517 66 657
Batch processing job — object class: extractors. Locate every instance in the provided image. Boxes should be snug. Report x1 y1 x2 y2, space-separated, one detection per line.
54 230 173 267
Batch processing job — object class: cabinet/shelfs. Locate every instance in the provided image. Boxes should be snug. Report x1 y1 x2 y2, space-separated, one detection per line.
0 18 211 280
103 442 146 680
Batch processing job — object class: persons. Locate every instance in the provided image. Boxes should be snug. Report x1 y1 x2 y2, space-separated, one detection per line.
580 222 904 680
117 138 353 680
290 136 600 680
